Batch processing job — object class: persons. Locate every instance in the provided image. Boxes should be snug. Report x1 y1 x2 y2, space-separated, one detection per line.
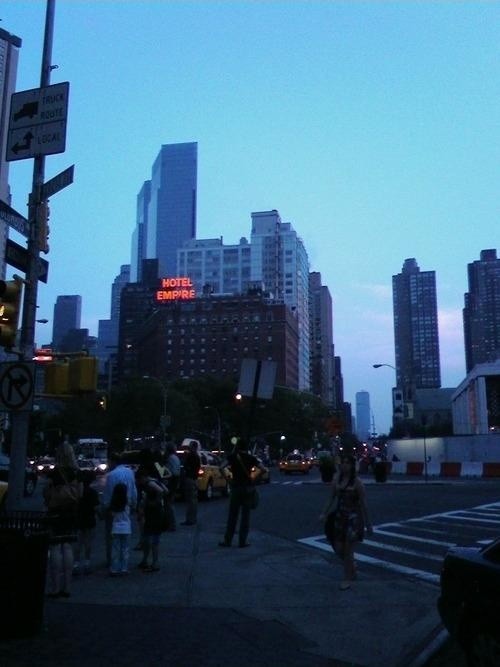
39 431 203 598
218 435 269 550
316 452 374 592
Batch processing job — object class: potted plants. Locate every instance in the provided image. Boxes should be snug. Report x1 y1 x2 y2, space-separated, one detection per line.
319 452 334 482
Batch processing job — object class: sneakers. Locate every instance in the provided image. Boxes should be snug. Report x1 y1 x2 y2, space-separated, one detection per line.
180 519 197 525
138 562 159 571
340 580 351 592
108 569 127 576
219 538 249 548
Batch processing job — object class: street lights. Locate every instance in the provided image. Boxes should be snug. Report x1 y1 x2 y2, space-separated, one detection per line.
141 374 170 455
373 363 428 481
205 405 221 450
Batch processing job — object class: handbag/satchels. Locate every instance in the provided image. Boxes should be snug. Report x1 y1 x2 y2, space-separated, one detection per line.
111 483 128 512
143 501 176 534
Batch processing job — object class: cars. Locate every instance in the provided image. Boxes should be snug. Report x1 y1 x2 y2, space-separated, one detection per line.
278 449 321 474
436 533 499 657
27 453 56 475
224 454 269 482
175 450 232 500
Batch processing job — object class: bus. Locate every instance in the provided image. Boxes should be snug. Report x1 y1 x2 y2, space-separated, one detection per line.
76 435 109 474
